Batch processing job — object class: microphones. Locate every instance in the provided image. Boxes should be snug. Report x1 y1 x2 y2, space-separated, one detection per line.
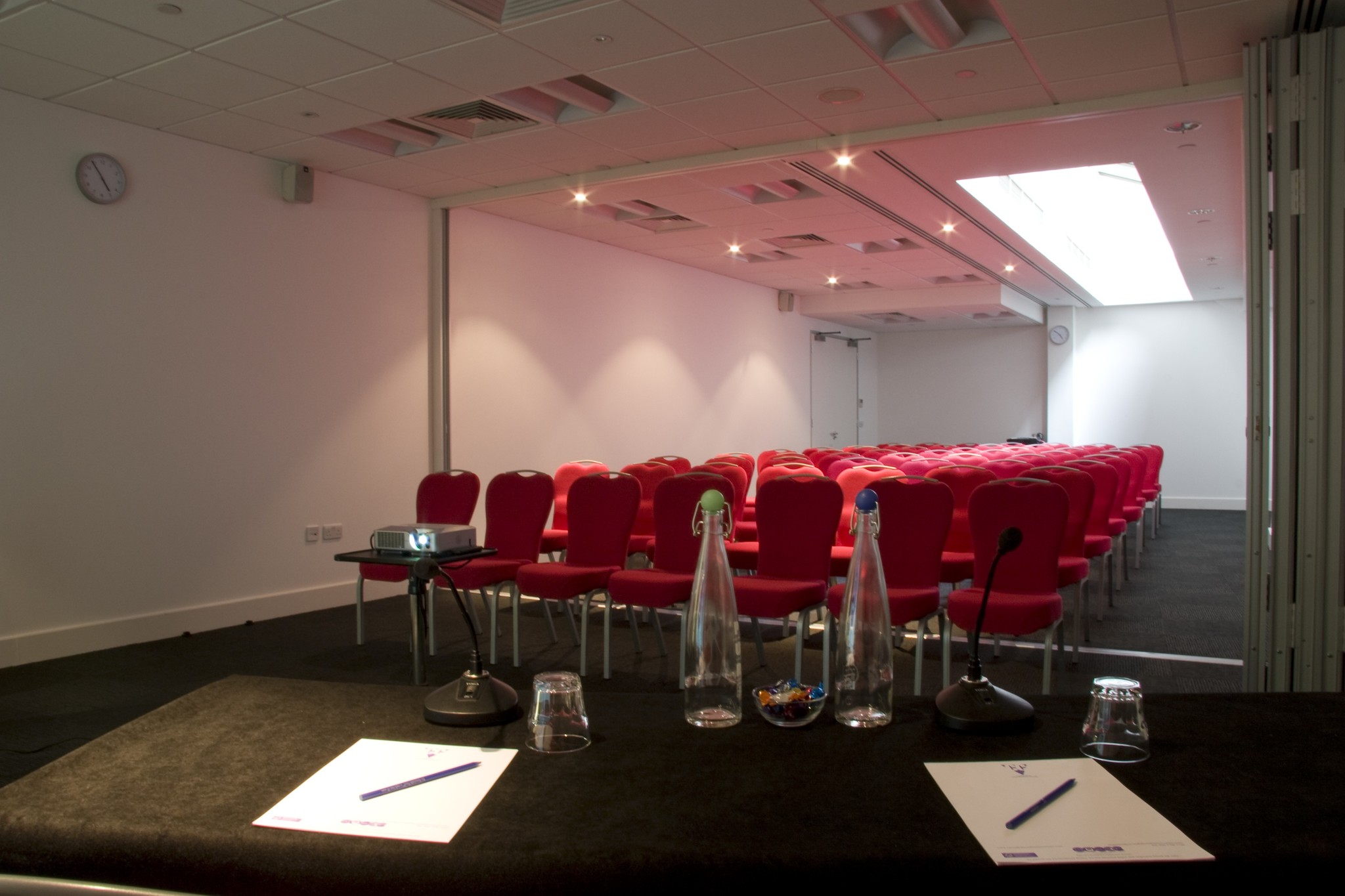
412 557 519 725
936 526 1036 719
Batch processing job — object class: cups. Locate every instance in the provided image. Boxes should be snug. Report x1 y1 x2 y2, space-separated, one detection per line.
525 669 591 754
1079 676 1151 764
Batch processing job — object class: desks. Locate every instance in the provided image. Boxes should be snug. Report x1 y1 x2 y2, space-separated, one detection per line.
1 670 1345 896
334 545 498 691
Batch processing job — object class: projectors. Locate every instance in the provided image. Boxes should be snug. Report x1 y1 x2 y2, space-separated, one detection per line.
374 523 477 557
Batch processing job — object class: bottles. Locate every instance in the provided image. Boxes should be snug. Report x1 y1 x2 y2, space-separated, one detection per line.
834 508 895 729
682 508 743 728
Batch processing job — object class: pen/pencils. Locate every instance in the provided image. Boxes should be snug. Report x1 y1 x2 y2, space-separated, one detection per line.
359 759 481 801
1006 779 1078 830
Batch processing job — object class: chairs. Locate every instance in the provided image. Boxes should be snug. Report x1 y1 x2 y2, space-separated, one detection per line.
340 441 1166 694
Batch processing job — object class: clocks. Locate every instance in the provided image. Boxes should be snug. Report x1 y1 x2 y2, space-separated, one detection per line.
1049 325 1070 345
73 152 128 203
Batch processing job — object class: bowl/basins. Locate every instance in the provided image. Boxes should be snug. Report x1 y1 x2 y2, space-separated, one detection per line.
752 685 828 728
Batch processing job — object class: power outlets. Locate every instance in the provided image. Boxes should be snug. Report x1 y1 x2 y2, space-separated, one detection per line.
321 524 343 540
305 525 320 541
